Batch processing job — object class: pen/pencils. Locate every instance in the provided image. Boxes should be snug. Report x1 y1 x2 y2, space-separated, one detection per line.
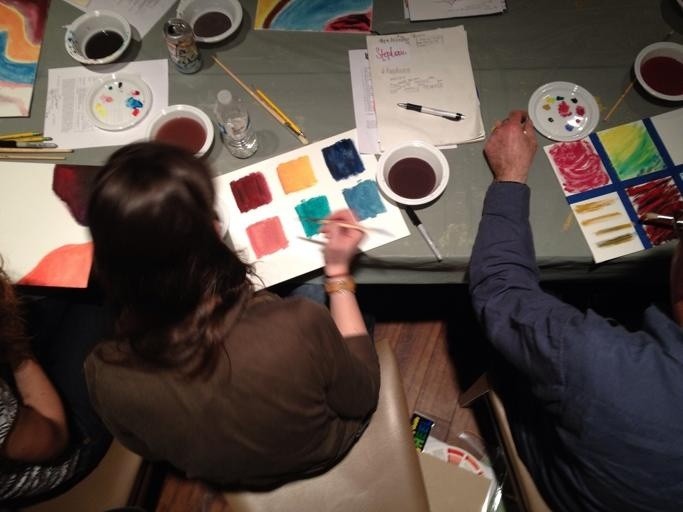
406 206 444 262
604 77 637 120
256 89 305 137
398 104 468 122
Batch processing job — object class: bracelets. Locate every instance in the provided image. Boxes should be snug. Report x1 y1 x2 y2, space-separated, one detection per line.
320 279 360 293
323 272 352 278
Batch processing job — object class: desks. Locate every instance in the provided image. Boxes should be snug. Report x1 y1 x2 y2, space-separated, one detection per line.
0 1 682 286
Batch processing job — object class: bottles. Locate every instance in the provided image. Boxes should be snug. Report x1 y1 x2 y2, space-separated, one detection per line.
213 89 259 159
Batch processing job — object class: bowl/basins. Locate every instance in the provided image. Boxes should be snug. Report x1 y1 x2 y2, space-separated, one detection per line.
64 10 131 67
144 104 215 162
376 140 450 207
634 41 683 101
177 0 243 43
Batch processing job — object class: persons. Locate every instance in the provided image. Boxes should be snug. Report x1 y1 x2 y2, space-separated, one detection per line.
468 106 681 510
0 259 105 504
79 147 381 492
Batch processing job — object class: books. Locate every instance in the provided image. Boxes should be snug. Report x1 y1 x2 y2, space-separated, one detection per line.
400 0 507 23
365 25 487 151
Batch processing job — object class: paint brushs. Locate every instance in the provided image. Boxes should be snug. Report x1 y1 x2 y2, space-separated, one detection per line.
644 212 681 221
302 218 390 236
0 131 74 160
210 54 309 144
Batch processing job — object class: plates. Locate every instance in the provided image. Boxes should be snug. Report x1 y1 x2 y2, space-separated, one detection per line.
85 72 153 132
527 81 600 142
